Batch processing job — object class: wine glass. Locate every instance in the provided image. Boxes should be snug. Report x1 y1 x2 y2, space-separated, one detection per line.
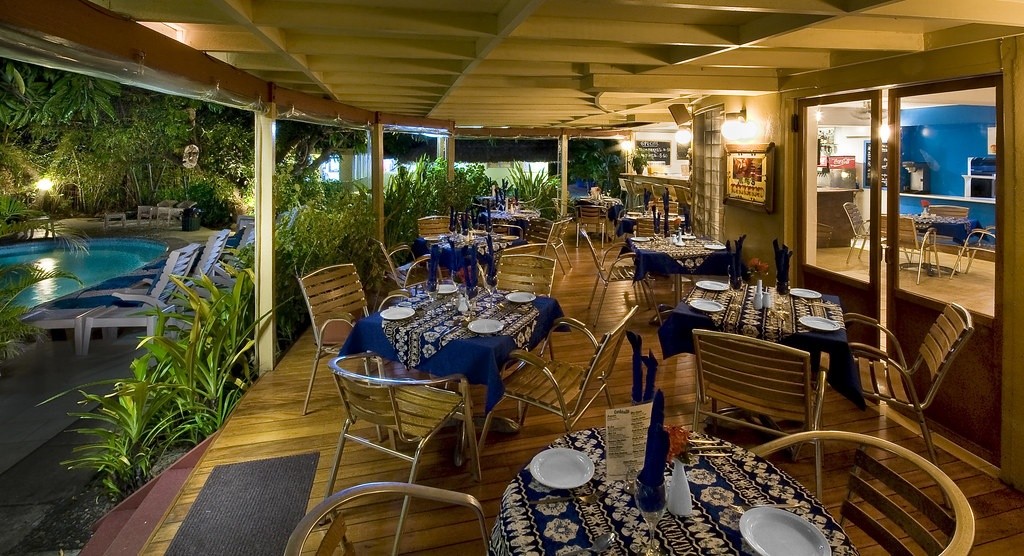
606 189 611 197
634 470 666 556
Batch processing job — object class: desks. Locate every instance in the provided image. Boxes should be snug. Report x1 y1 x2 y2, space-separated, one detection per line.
902 213 991 273
480 208 540 237
617 234 750 324
574 195 623 234
423 231 528 286
338 282 572 469
616 211 685 238
488 424 860 556
475 196 508 207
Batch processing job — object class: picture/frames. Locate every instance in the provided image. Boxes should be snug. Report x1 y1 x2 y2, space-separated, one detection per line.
722 143 776 214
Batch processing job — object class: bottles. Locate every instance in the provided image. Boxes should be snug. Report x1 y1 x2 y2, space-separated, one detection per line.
647 163 653 174
753 293 762 310
458 298 468 312
762 292 771 308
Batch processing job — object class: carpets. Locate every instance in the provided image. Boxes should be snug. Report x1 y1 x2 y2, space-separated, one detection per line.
163 449 320 556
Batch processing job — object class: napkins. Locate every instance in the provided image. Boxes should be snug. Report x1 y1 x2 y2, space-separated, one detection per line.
495 179 519 209
427 234 498 297
638 390 666 511
625 331 642 403
448 200 492 235
642 349 659 399
725 234 748 284
773 238 794 294
643 186 690 238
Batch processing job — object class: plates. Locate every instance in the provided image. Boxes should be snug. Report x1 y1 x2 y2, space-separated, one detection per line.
704 244 726 249
631 237 649 241
696 281 730 290
529 448 594 489
690 300 724 311
380 308 415 320
789 287 822 298
677 235 696 239
799 316 840 330
437 285 457 293
505 292 536 302
739 507 831 556
467 319 504 333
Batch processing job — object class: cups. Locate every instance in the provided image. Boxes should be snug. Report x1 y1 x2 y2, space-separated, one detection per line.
900 213 911 218
931 213 937 221
683 226 692 235
681 165 689 177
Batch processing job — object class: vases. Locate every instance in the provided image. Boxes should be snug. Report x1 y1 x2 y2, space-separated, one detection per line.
675 229 686 246
667 457 693 516
922 206 928 213
753 280 764 310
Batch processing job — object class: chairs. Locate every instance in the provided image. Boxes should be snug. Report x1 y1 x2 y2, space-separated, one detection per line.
38 199 299 312
301 179 977 556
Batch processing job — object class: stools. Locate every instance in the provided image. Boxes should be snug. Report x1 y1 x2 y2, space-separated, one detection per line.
104 212 127 230
20 305 106 355
83 303 176 358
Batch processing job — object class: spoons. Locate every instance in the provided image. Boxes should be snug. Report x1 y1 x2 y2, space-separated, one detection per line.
561 532 615 556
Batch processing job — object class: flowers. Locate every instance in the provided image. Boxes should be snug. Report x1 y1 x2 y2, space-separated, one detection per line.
666 425 692 464
748 256 769 281
673 218 682 227
920 199 929 207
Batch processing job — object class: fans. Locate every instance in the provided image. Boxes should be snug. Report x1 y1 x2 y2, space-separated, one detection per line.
848 101 889 121
